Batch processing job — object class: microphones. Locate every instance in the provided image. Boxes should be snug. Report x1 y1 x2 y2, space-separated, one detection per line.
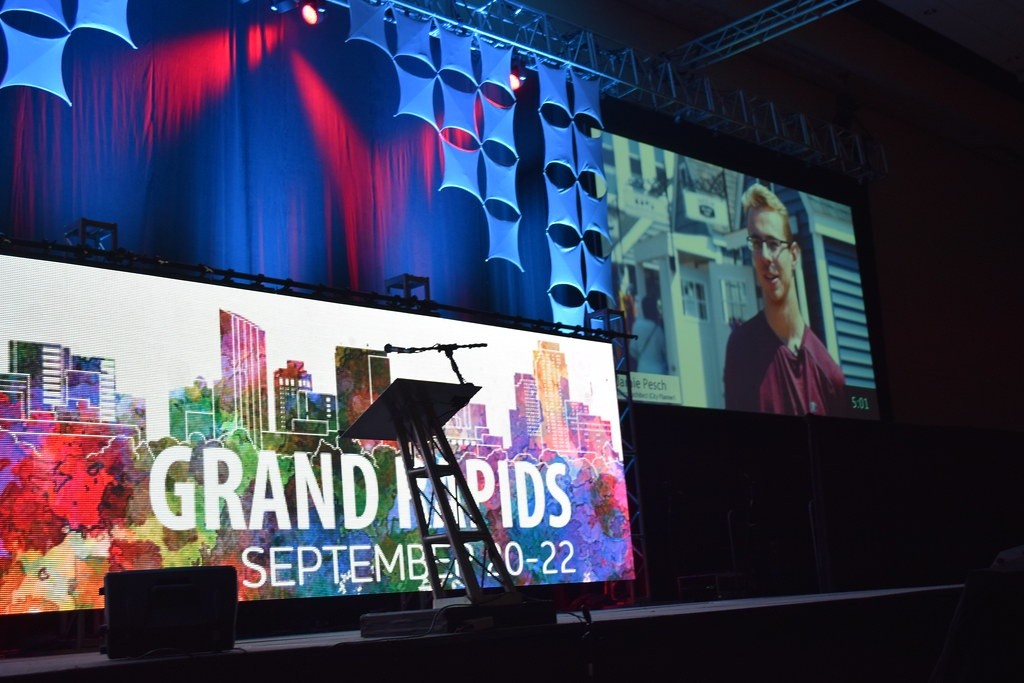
385 344 408 354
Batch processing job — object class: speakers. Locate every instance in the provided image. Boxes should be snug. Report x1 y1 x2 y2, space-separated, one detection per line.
97 566 238 659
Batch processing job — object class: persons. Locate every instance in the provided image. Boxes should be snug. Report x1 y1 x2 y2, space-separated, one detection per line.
628 294 667 375
725 182 847 417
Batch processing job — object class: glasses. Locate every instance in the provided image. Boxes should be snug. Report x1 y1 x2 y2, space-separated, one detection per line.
747 234 791 250
